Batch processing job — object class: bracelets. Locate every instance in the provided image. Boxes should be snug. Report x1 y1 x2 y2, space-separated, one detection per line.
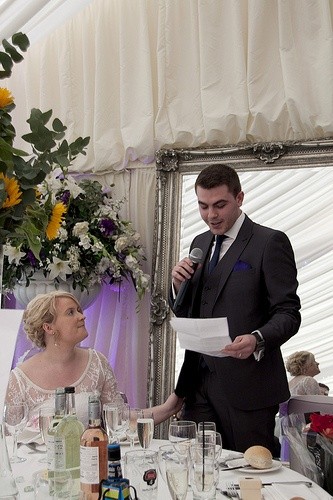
149 408 154 418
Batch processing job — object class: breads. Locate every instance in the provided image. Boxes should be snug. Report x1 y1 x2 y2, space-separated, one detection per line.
244 446 273 470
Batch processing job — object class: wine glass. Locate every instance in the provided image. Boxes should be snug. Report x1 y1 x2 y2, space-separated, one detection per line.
3 402 30 464
101 402 154 470
39 406 54 463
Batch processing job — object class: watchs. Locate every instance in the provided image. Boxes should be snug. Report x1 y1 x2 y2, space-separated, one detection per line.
252 331 264 351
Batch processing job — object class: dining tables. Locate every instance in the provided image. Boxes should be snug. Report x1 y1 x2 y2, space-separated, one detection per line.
115 439 333 499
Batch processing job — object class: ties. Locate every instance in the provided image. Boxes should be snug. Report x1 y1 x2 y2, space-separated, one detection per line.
208 235 230 276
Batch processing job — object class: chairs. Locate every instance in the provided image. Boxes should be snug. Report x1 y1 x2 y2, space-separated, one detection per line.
287 395 333 484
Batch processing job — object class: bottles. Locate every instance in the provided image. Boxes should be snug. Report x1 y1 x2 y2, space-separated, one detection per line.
47 384 131 500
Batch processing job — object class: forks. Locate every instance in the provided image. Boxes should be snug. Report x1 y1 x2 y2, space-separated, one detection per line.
225 481 239 500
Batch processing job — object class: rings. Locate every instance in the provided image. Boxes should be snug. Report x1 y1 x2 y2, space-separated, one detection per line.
239 352 242 358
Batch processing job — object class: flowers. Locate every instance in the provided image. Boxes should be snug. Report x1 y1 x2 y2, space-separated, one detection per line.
0 32 151 298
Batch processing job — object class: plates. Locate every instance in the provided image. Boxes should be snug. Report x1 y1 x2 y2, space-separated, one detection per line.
226 458 283 474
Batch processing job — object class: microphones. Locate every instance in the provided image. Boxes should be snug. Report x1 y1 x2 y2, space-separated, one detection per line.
172 248 203 313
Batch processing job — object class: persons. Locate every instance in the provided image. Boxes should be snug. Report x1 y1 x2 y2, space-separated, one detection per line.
6 291 184 436
169 163 329 460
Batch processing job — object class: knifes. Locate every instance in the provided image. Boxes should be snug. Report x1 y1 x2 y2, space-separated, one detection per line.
221 464 250 472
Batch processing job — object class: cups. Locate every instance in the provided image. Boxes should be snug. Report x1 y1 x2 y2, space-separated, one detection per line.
123 420 222 500
34 467 87 500
